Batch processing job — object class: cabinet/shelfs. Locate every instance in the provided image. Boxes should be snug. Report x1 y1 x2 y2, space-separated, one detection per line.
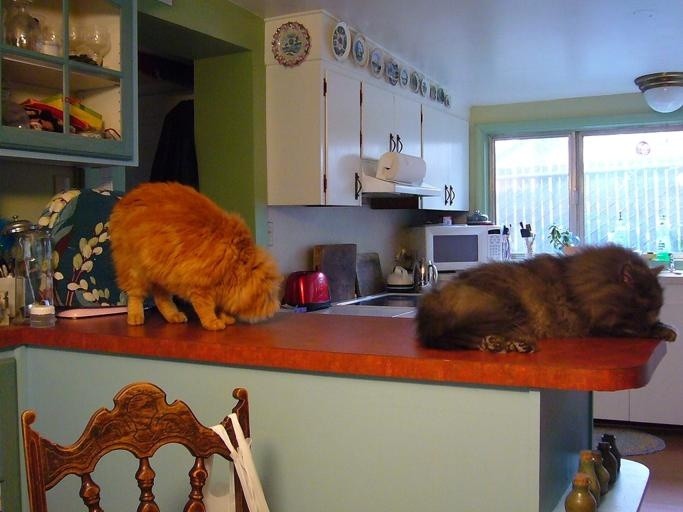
362 81 422 163
1 0 139 168
591 276 683 433
265 59 363 207
371 101 470 212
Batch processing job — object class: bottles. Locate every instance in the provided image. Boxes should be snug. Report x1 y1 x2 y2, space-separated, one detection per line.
2 214 58 326
563 432 623 512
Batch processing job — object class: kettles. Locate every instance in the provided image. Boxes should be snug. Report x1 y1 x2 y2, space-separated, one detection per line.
413 258 438 292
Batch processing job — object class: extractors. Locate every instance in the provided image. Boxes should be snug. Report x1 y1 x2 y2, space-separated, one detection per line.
360 173 441 198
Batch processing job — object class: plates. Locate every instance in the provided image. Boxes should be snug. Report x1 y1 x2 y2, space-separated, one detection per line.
420 78 428 97
385 61 398 86
409 71 420 93
444 95 451 106
271 22 310 69
331 23 351 61
429 85 436 100
436 88 444 103
370 47 385 79
400 70 408 87
350 32 369 67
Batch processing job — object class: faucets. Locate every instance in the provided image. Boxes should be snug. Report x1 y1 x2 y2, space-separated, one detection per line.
667 252 675 272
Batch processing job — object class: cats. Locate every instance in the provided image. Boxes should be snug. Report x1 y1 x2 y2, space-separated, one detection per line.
413 244 678 354
109 182 285 332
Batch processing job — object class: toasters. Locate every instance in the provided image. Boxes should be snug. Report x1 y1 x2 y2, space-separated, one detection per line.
281 270 331 311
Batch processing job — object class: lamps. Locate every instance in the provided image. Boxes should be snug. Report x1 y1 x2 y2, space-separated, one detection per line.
634 72 683 113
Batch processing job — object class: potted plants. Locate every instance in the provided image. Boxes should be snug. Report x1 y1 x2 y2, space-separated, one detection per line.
547 221 581 256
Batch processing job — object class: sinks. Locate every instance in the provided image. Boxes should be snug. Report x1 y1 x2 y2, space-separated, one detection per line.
336 292 424 307
660 273 683 276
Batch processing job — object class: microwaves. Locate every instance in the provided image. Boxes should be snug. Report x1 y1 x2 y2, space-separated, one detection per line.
408 224 503 272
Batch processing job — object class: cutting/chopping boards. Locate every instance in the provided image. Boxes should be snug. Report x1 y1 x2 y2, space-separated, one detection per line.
353 250 384 297
311 242 356 301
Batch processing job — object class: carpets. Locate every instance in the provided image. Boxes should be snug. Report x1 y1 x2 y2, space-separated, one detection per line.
591 426 667 455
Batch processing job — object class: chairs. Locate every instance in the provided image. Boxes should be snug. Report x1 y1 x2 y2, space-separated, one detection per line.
21 383 250 511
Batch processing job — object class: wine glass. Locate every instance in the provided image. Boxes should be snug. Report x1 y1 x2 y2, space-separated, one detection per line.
4 0 43 52
86 29 111 65
